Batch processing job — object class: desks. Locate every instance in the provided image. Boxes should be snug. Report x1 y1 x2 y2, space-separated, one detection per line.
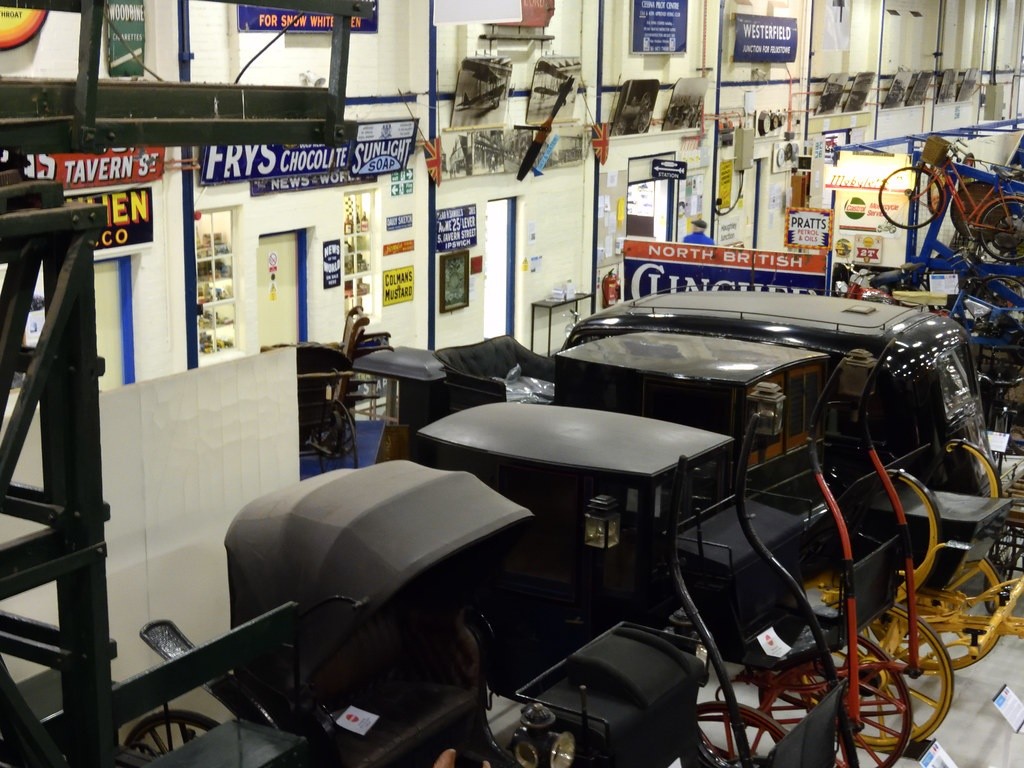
531 292 593 357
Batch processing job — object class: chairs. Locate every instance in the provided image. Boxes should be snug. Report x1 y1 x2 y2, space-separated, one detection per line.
325 305 370 401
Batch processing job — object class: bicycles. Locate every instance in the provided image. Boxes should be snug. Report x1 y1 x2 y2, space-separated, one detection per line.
879 133 1024 263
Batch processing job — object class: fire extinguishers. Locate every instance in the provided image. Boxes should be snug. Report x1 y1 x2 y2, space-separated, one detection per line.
601 267 621 309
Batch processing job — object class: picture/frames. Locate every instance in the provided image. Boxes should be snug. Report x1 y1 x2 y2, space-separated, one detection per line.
440 250 469 314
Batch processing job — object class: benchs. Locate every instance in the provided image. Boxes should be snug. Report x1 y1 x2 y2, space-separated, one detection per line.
740 435 885 542
302 609 493 768
